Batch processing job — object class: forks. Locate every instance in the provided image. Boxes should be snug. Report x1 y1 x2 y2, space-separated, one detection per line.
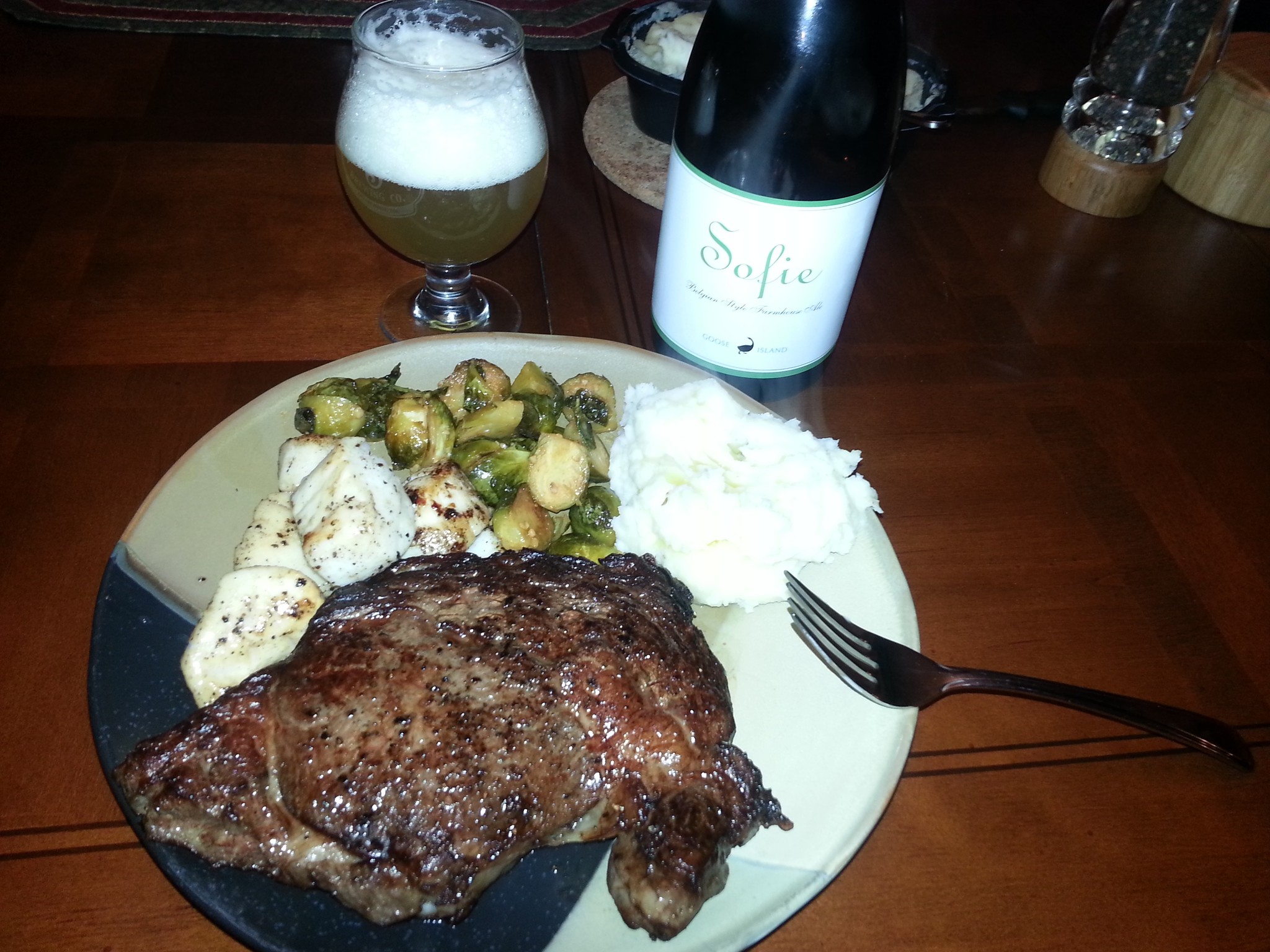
783 570 1253 768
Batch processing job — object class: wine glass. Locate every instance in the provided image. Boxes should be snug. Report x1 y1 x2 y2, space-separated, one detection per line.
334 0 551 341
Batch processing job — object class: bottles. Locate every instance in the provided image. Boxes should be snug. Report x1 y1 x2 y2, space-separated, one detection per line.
649 0 916 399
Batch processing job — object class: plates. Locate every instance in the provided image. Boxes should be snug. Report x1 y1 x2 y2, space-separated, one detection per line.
87 332 917 952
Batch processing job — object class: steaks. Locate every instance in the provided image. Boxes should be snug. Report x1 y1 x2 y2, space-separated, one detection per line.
111 544 798 944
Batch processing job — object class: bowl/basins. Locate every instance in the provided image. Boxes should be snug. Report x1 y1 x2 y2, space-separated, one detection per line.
603 4 963 169
